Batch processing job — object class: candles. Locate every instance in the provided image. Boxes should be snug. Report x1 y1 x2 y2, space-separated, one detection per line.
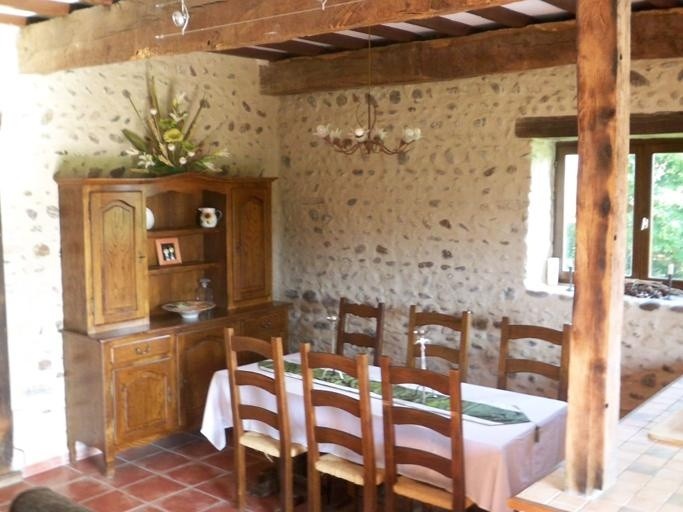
547 256 559 287
668 263 673 274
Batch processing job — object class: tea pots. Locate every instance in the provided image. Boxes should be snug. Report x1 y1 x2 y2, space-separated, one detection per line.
198 206 223 229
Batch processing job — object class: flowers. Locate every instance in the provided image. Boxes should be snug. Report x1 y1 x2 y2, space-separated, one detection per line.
116 43 236 179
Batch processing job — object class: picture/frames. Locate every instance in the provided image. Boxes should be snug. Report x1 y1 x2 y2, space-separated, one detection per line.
154 237 183 267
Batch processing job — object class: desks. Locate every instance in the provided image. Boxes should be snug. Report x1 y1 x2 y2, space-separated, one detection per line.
218 353 569 512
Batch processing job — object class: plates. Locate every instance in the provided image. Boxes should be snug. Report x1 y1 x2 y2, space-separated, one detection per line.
160 301 216 319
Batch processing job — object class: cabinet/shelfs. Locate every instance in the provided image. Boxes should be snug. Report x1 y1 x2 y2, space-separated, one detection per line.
50 171 150 339
180 301 294 434
140 169 228 326
218 177 273 312
59 324 182 479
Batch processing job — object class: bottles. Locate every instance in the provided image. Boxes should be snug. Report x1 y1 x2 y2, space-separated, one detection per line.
145 206 155 230
195 278 215 306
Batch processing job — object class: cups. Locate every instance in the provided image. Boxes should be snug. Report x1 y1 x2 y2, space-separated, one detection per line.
545 255 560 288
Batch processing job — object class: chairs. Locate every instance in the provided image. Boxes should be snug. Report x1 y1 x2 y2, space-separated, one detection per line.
405 305 473 382
379 356 476 512
223 328 308 511
301 340 387 511
336 297 386 368
496 317 571 405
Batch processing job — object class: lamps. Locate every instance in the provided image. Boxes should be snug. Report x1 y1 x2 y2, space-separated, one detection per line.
314 22 427 158
